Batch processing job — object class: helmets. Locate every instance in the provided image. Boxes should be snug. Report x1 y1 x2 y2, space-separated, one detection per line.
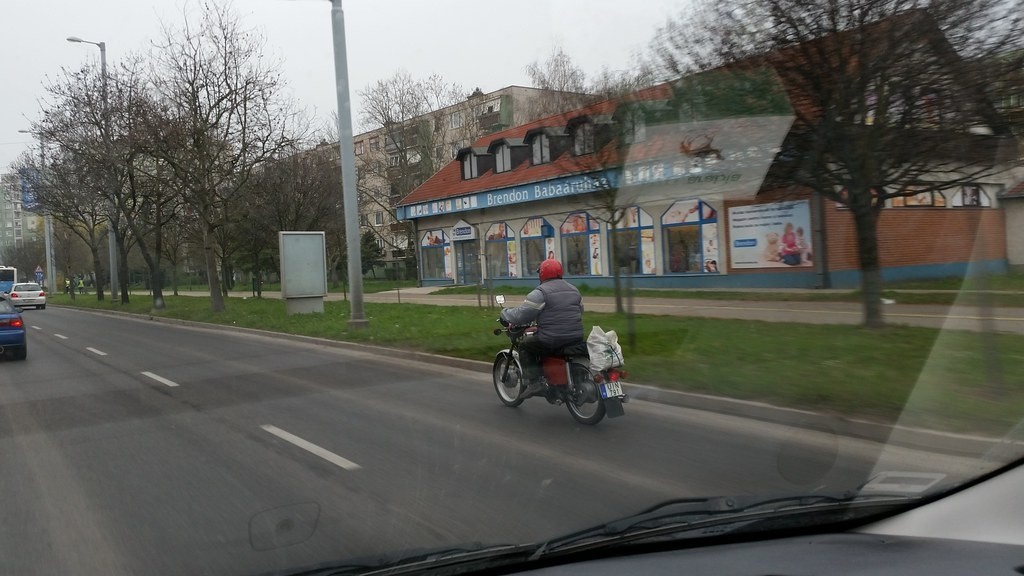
536 259 563 282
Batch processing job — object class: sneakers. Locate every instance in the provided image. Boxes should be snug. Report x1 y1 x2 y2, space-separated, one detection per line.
520 382 543 398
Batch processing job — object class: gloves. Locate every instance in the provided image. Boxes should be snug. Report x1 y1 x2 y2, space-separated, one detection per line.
500 316 509 327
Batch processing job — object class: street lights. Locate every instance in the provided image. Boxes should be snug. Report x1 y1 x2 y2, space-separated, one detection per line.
19 129 58 297
66 36 119 303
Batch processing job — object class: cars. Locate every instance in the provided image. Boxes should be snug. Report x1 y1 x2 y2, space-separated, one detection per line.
0 299 27 360
6 282 46 310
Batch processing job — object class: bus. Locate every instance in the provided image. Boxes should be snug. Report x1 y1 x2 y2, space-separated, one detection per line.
0 266 17 301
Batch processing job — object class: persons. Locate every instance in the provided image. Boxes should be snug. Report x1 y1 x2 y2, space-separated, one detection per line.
501 260 584 400
64 277 70 294
78 278 84 295
427 203 812 278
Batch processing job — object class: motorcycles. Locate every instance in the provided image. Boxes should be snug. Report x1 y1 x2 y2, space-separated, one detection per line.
492 294 629 426
